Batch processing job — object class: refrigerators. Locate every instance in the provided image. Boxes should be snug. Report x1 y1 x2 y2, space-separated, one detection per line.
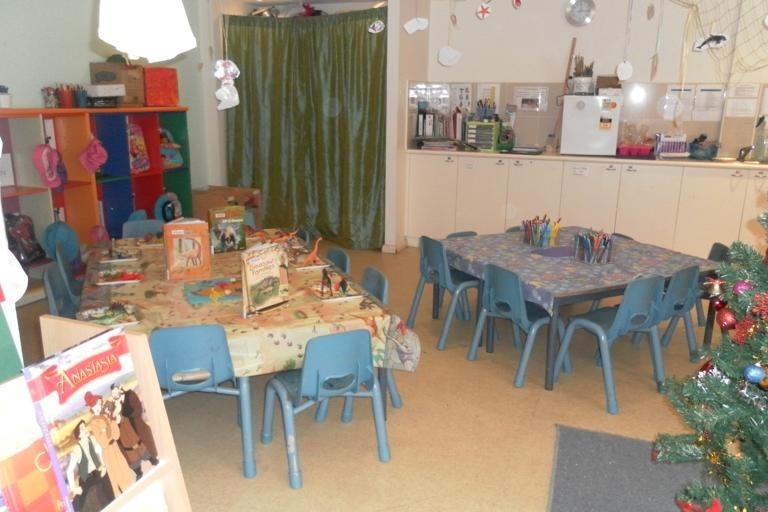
558 93 623 157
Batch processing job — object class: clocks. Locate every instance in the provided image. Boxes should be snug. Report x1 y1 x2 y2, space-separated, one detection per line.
565 0 597 26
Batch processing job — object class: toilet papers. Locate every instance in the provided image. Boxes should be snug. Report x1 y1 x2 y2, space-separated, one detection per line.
0 92 13 109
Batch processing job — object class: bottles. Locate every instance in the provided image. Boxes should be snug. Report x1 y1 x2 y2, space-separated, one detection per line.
495 111 516 153
44 86 57 108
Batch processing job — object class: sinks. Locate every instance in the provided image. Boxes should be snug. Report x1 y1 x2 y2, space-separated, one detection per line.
712 158 762 164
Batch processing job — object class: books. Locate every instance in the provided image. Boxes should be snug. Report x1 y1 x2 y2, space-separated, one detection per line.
163 217 212 283
300 270 362 304
240 243 287 320
288 244 325 272
206 205 246 253
94 266 145 286
96 247 142 265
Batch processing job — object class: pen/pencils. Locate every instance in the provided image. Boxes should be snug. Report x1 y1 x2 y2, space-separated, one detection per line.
55 83 72 91
574 226 613 265
522 214 562 246
585 62 593 72
575 56 583 72
477 98 496 109
73 84 88 92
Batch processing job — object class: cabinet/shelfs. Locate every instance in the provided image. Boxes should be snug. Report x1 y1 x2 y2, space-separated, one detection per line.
561 159 682 253
0 106 193 309
683 166 768 259
458 157 562 239
404 155 458 248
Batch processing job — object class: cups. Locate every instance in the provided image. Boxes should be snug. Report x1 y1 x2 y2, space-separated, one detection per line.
0 94 12 108
57 89 88 109
573 76 593 93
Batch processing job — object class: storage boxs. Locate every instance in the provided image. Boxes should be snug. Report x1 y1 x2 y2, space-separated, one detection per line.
88 58 181 108
190 185 262 229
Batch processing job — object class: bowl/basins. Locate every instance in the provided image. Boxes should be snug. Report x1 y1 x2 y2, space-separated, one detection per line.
689 143 719 161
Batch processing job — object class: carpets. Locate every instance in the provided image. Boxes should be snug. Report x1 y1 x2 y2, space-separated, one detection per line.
544 423 741 511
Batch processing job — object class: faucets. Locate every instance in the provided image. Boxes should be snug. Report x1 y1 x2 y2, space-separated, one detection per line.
736 145 756 162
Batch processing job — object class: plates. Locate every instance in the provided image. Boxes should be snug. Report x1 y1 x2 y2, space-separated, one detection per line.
95 270 141 286
97 250 139 264
512 146 546 155
